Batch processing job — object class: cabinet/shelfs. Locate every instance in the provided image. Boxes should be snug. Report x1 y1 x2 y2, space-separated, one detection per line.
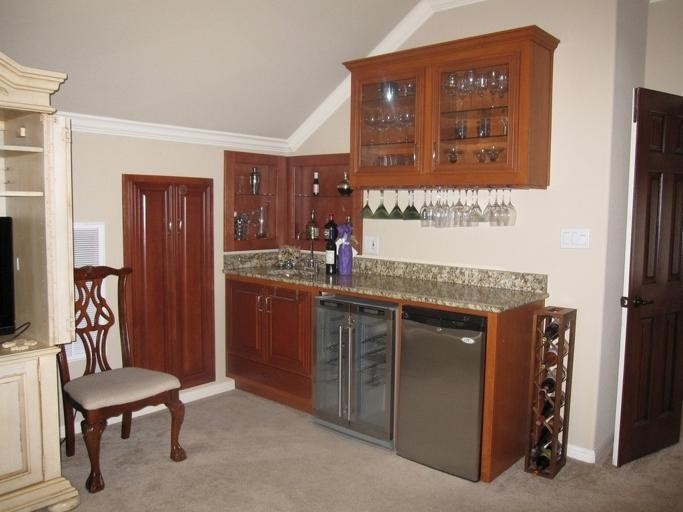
294 192 354 241
120 172 215 390
222 279 312 399
524 303 578 478
0 146 43 200
235 189 275 241
342 24 560 191
0 330 82 510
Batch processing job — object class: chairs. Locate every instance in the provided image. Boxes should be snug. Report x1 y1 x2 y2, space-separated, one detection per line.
51 264 185 493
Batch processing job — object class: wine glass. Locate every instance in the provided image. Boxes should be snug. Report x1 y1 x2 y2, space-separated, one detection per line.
443 69 507 112
355 182 517 229
361 107 414 146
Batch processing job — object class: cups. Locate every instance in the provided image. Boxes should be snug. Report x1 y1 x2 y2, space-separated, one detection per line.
364 152 415 166
445 145 504 164
455 116 509 141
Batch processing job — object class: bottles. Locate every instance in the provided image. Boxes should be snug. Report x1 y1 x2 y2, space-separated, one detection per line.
234 166 352 239
531 315 570 475
324 225 353 275
